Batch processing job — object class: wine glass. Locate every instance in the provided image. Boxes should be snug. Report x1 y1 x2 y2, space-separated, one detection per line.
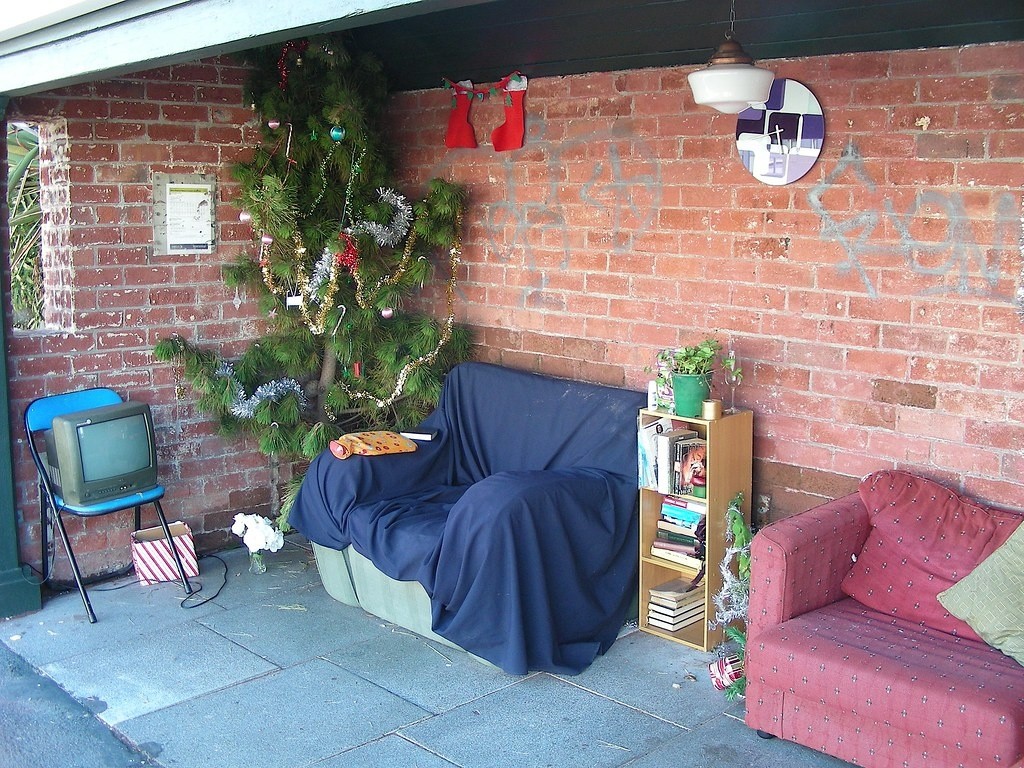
722 350 742 414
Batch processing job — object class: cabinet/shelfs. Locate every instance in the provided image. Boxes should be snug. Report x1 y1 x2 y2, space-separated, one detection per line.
636 403 755 653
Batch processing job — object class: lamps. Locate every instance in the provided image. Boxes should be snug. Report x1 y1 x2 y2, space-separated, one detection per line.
686 0 775 115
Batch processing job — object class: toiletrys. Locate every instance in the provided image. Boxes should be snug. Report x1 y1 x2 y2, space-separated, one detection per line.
647 379 659 410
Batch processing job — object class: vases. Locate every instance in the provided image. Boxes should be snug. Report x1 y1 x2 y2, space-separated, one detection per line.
247 546 269 576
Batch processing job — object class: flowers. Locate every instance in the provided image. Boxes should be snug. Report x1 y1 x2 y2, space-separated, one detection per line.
232 512 285 554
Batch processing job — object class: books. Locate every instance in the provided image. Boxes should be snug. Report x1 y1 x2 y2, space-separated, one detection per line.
650 496 705 571
636 417 707 497
399 426 439 441
647 576 705 632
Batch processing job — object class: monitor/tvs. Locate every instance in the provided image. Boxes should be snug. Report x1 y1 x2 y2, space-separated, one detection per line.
43 401 157 506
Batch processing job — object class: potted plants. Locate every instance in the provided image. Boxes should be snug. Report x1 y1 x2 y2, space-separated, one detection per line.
644 326 744 416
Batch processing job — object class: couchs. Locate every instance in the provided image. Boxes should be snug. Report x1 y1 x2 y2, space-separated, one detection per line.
745 470 1024 768
288 362 651 668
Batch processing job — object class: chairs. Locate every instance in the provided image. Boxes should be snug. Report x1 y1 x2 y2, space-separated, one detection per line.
23 386 192 623
